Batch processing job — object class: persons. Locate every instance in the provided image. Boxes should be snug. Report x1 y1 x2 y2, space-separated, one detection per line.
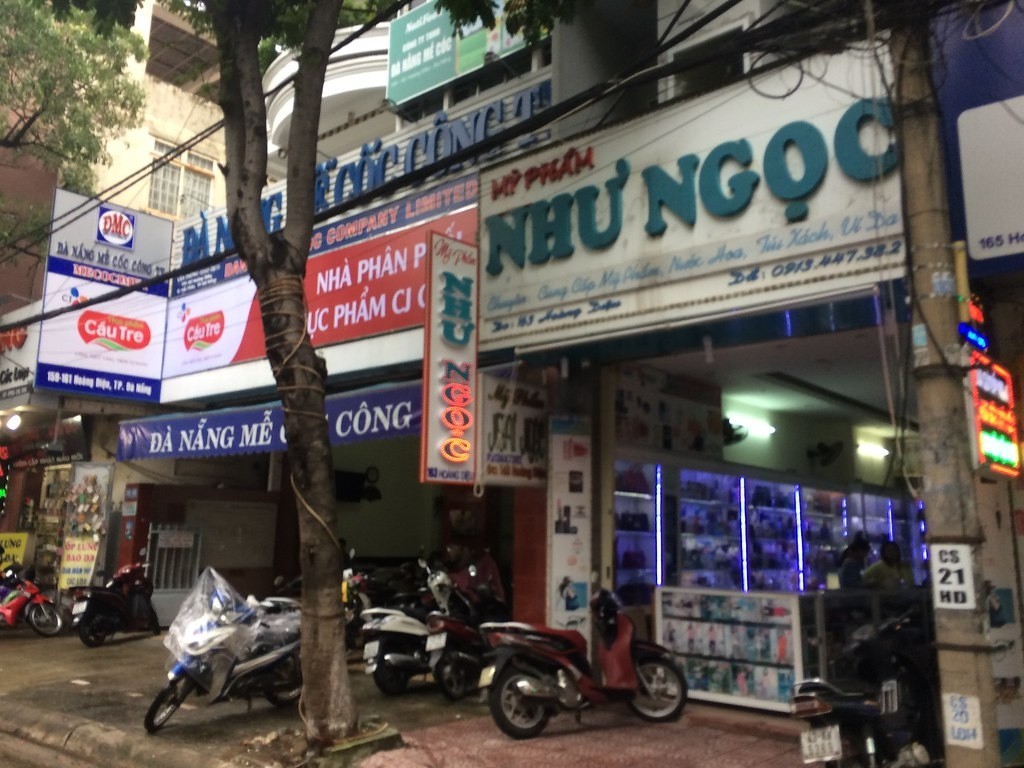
863 542 915 588
839 538 871 586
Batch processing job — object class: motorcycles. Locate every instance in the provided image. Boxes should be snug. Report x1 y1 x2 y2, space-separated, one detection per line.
69 547 161 648
475 564 689 741
142 564 304 734
274 547 429 662
359 588 435 695
418 557 489 701
787 605 945 768
0 555 65 637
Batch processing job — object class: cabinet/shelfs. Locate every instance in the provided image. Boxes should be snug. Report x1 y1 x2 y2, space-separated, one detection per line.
614 442 928 615
